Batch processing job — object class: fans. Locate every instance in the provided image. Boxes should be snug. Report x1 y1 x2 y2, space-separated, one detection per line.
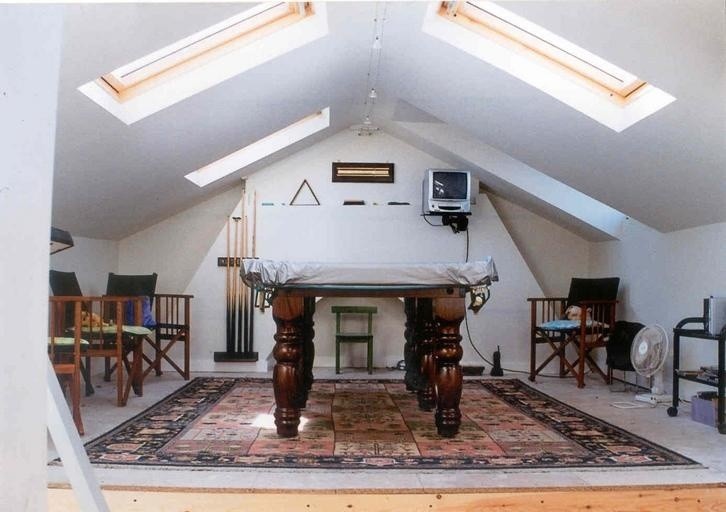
629 323 674 405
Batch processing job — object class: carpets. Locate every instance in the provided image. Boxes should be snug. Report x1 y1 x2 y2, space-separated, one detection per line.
47 374 710 472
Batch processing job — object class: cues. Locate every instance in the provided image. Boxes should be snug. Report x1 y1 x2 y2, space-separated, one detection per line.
227 189 256 354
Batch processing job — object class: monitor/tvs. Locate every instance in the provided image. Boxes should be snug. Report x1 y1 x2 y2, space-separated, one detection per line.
423 169 471 215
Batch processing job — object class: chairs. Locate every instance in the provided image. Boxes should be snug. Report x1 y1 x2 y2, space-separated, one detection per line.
331 305 378 375
48 268 195 435
527 275 620 390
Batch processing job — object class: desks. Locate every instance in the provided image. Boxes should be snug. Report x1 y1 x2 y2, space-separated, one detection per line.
240 256 498 439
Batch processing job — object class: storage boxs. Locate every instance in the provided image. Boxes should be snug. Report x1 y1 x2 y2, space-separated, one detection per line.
692 395 718 428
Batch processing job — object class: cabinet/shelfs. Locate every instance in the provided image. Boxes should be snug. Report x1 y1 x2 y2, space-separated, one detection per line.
672 316 726 423
